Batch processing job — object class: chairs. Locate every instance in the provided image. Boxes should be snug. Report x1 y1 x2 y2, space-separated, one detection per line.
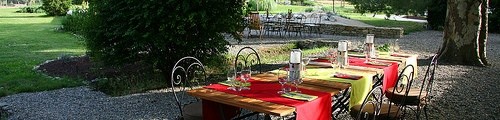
171 11 438 120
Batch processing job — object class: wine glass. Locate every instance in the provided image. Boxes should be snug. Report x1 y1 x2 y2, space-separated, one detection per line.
225 65 252 91
277 69 302 94
330 55 349 76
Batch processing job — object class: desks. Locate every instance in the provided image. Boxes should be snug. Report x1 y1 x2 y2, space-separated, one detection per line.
185 47 417 120
